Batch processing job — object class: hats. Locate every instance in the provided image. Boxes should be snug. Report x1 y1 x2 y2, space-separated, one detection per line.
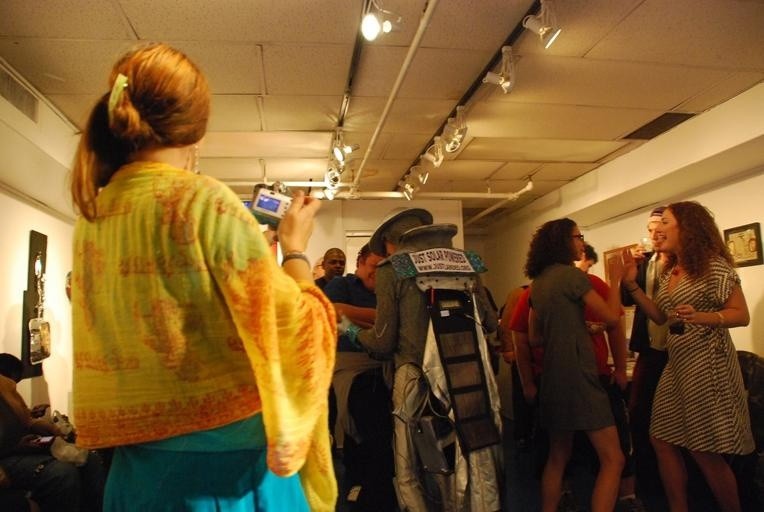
369 206 433 258
650 206 667 221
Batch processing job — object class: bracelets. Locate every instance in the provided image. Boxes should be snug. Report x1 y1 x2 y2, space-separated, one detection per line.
281 251 311 265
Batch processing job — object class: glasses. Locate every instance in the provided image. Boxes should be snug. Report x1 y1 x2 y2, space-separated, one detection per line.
573 234 585 242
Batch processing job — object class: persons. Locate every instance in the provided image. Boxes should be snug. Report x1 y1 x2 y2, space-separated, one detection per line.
313 207 505 512
497 217 624 512
620 202 754 509
68 42 338 512
1 352 112 512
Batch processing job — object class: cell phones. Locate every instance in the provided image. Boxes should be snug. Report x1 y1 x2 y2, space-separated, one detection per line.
40 436 53 445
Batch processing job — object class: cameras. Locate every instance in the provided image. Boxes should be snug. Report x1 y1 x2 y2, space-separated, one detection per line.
250 181 293 220
51 409 76 444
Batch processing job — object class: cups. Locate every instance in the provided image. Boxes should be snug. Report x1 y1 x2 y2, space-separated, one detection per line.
639 237 655 260
667 308 688 336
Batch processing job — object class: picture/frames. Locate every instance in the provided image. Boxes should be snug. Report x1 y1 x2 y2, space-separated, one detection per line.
604 244 638 283
723 223 764 268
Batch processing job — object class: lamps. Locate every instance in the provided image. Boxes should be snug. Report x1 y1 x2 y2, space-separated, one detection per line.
396 0 562 201
361 0 402 41
323 127 359 201
